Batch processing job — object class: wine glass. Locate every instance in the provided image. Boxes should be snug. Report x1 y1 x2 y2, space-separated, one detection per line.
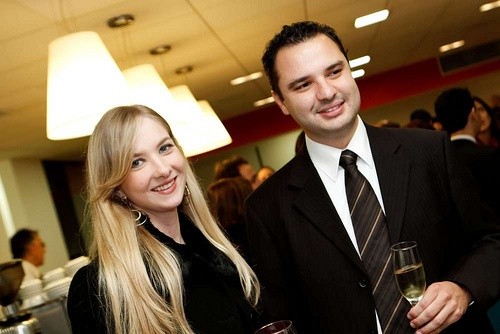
390 241 426 308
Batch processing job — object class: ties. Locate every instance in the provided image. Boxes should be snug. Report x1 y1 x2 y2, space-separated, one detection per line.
339 150 417 334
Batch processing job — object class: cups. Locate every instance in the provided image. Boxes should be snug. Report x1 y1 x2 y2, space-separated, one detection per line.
19 255 89 300
256 320 295 334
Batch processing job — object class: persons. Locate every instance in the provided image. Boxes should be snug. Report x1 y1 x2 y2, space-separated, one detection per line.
214 158 274 193
207 177 245 225
66 105 260 334
240 22 500 334
9 227 46 280
472 96 500 147
432 85 500 220
380 109 445 131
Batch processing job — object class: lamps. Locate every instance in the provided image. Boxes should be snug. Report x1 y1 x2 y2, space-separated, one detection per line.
46 0 132 141
176 64 233 156
107 15 176 147
151 44 199 158
